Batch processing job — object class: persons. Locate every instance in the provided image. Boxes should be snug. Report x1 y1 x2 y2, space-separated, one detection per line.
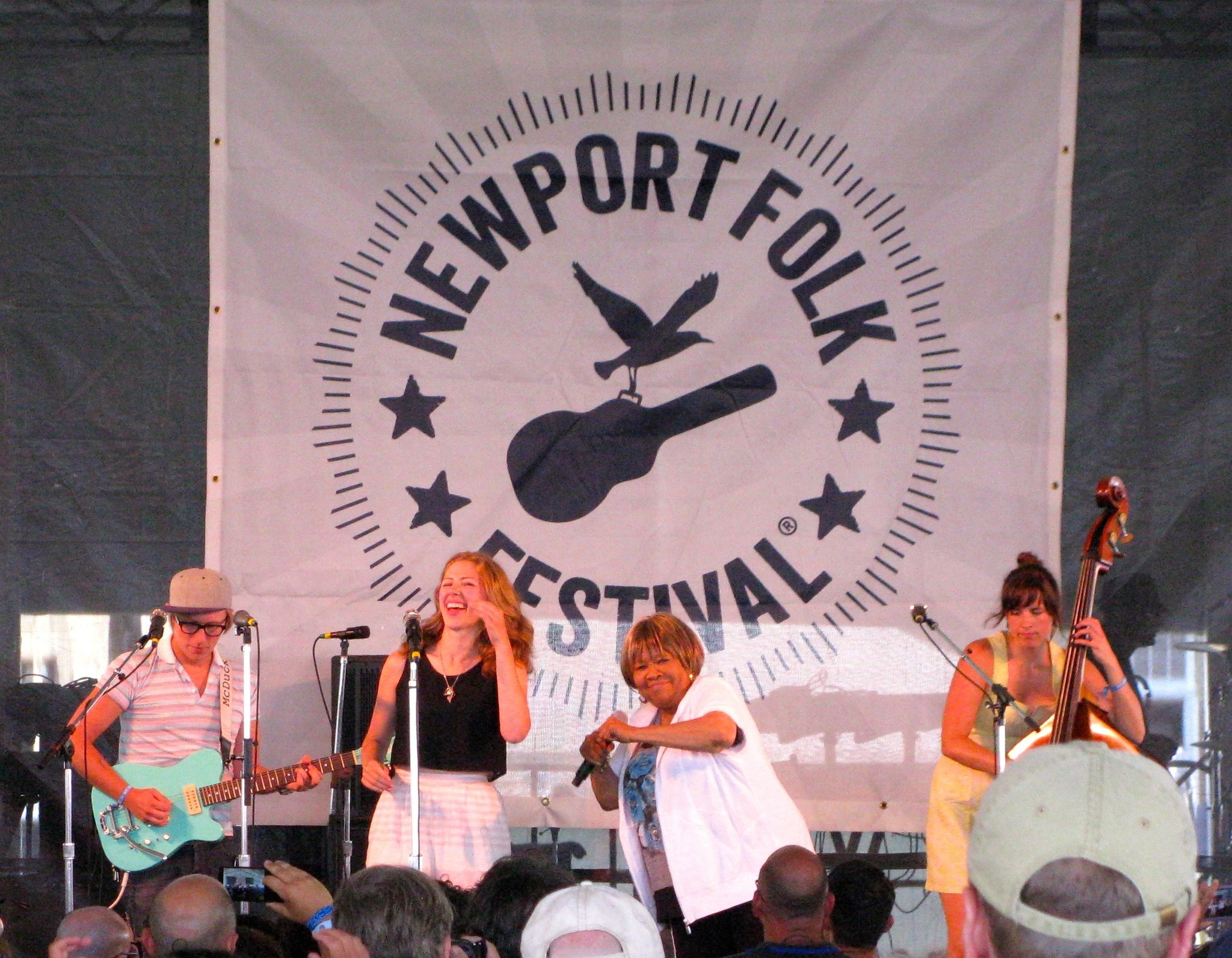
361 552 534 890
65 567 323 945
1198 879 1232 958
0 850 666 958
723 845 850 958
823 860 895 958
579 612 819 958
925 551 1146 958
967 741 1202 958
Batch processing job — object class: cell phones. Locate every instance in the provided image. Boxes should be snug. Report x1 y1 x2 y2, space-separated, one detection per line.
219 866 280 902
1201 884 1232 922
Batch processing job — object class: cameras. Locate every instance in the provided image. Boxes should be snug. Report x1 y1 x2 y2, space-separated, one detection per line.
449 938 488 958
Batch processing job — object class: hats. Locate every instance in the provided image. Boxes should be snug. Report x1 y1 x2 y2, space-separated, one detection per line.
154 567 231 613
517 880 666 958
963 739 1200 941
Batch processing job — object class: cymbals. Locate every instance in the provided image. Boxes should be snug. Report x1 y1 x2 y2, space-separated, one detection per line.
1173 642 1230 654
1188 738 1232 754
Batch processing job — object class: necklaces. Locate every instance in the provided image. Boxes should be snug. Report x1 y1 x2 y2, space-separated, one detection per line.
439 641 469 703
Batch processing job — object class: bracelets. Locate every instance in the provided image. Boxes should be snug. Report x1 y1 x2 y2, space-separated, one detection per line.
1108 677 1127 691
307 904 334 931
593 753 610 772
313 919 332 932
117 785 132 804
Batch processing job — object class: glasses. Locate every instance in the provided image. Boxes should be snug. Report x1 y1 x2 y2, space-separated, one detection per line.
175 613 227 636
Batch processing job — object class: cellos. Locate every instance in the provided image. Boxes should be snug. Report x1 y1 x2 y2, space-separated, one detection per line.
1003 475 1169 768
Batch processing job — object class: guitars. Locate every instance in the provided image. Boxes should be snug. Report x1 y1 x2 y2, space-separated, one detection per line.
92 733 394 875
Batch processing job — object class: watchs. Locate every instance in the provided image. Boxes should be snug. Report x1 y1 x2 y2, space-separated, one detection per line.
279 786 293 796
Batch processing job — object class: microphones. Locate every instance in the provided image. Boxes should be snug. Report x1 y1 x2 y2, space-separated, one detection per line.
403 609 421 662
319 626 370 640
572 711 627 788
233 610 258 627
911 604 926 623
149 608 166 648
